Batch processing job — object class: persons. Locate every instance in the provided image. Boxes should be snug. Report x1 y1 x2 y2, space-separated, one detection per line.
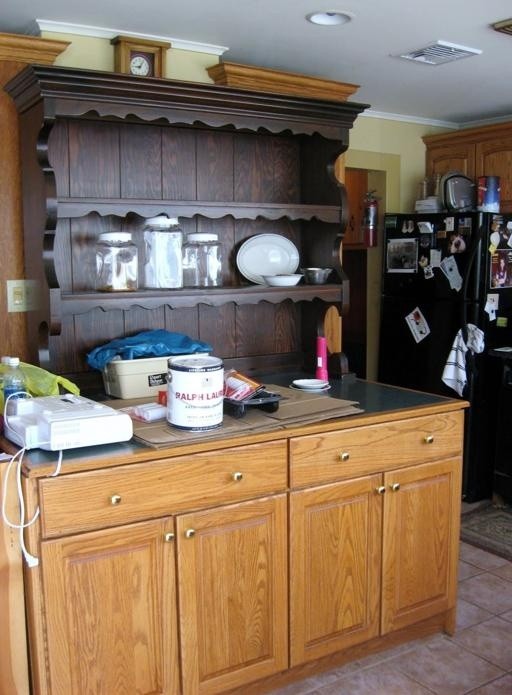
494 256 508 289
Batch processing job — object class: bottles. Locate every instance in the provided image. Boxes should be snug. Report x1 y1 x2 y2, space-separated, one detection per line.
0 357 14 408
92 231 138 293
3 359 26 404
139 216 184 290
185 233 223 287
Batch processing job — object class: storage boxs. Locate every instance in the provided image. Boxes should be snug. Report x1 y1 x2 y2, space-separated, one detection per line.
101 351 215 399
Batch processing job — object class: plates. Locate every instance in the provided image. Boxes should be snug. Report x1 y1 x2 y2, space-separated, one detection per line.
293 378 328 388
289 384 331 394
236 232 300 286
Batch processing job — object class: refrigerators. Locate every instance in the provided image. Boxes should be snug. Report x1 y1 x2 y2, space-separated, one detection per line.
378 210 512 503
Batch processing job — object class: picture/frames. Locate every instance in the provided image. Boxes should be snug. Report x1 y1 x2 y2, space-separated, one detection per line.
487 256 509 289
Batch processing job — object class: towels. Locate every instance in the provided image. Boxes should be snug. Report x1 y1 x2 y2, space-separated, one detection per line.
441 321 487 399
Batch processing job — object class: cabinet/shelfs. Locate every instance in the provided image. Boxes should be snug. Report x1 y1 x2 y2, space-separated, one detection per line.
423 120 512 213
289 397 471 685
20 429 292 693
5 62 374 404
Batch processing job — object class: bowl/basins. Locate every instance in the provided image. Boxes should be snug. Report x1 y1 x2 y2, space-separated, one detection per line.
261 273 304 288
300 266 333 285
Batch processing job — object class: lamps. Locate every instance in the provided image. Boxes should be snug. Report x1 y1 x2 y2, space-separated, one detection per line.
305 9 358 29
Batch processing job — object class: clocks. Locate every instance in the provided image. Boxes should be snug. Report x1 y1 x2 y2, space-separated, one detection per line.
110 34 174 81
